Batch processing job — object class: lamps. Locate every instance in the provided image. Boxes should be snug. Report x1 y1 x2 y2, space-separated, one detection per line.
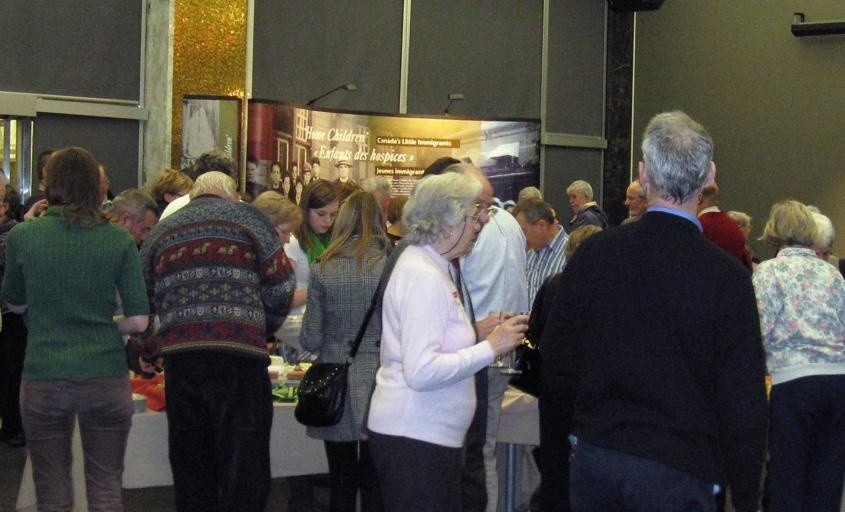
307 83 358 107
444 93 465 115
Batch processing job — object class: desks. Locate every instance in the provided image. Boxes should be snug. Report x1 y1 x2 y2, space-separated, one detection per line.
121 314 541 511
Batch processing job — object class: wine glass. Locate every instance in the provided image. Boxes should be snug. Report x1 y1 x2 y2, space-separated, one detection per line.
487 310 523 374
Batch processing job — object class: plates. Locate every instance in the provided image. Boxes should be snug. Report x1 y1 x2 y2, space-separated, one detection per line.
132 392 148 413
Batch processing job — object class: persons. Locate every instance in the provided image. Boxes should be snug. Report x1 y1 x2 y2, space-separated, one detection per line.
563 222 602 262
751 200 845 512
565 179 610 229
538 110 769 509
246 154 357 207
726 210 752 239
1 145 568 511
621 178 647 224
698 178 745 262
806 205 845 281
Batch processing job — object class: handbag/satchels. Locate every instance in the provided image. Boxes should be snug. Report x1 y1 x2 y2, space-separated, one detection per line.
294 362 348 427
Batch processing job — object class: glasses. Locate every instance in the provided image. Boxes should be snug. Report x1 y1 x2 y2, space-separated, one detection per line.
475 203 498 217
464 212 481 224
